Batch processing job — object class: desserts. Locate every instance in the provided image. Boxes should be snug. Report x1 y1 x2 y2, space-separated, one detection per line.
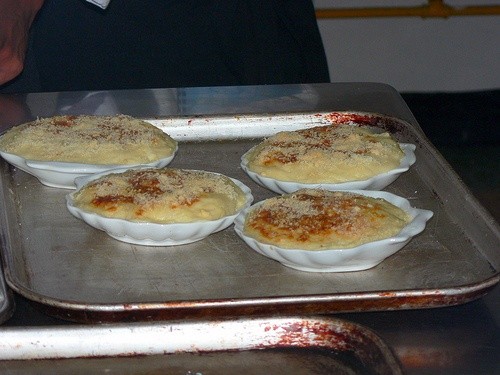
242 187 409 250
0 111 177 165
246 123 404 183
73 167 247 224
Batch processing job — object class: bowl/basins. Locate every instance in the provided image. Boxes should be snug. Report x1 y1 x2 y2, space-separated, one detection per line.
64 165 254 246
0 134 179 191
239 140 417 195
233 190 435 273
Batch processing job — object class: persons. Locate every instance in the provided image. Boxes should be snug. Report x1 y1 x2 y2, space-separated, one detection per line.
0 1 332 93
